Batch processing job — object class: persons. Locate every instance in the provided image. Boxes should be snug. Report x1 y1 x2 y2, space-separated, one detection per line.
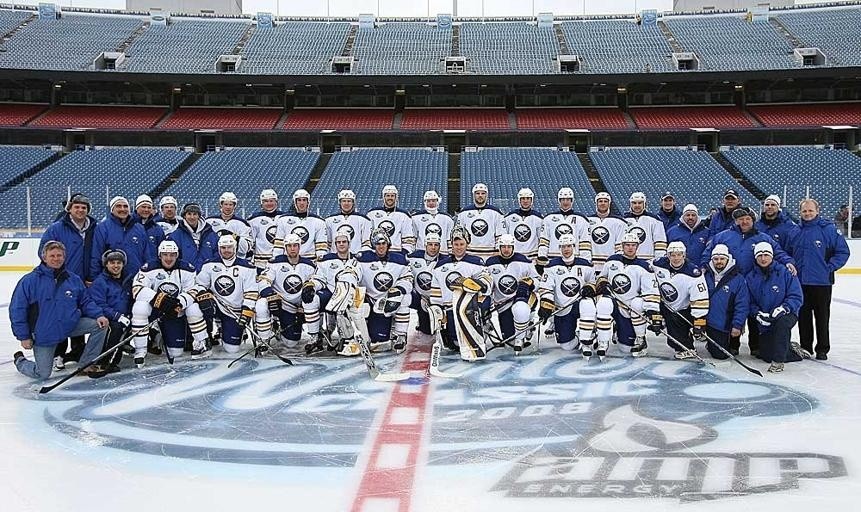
8 184 850 381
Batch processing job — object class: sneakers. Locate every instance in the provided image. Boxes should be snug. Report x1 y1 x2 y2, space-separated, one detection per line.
52 356 65 371
78 364 108 379
674 349 697 359
790 342 827 360
304 335 323 350
392 334 407 349
581 342 609 355
514 338 523 351
630 337 646 352
692 321 706 341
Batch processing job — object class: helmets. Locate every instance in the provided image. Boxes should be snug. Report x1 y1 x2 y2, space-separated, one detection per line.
260 189 278 213
424 233 441 246
293 188 310 208
382 185 398 199
158 239 179 259
594 192 612 208
218 235 237 260
472 183 488 201
517 188 534 206
629 192 646 209
219 192 237 216
284 234 301 249
558 188 574 204
621 233 640 251
423 191 439 214
558 234 576 249
338 190 356 206
668 242 687 260
370 229 392 249
160 196 177 212
334 231 351 248
498 234 515 259
450 226 471 244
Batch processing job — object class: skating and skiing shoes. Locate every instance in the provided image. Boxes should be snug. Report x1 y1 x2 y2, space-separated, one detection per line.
134 349 146 365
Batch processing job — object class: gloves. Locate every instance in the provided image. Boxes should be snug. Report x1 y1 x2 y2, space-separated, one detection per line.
650 315 662 333
300 285 313 302
158 291 214 316
755 304 790 326
581 278 612 296
538 300 555 323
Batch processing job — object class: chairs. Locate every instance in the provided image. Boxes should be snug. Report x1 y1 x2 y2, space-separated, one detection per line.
0 9 861 228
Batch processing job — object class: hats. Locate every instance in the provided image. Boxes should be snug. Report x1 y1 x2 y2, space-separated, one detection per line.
662 190 780 221
102 249 128 266
181 203 202 218
710 244 728 260
65 193 92 214
754 242 774 259
109 195 130 213
136 195 153 209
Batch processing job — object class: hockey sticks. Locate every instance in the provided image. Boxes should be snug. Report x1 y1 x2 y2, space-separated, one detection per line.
317 322 340 352
605 286 716 368
476 292 585 356
214 296 293 366
660 298 763 377
30 310 178 394
207 288 763 381
228 314 302 368
